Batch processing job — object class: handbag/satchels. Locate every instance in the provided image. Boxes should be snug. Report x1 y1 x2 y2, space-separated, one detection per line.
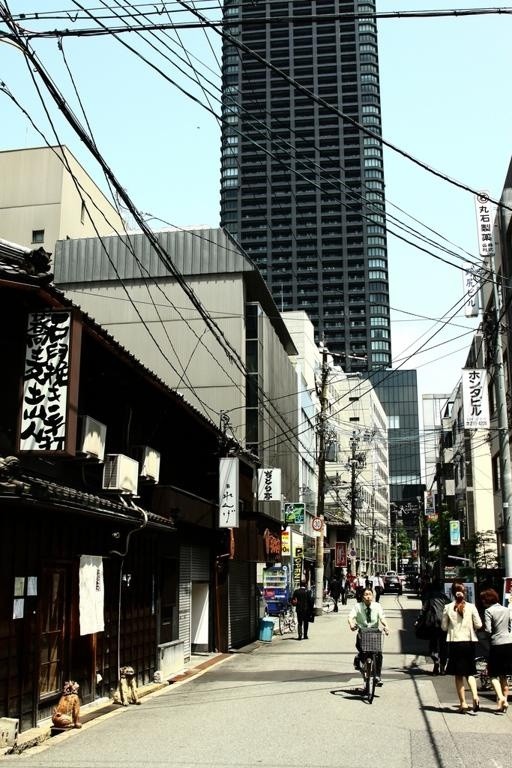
416 616 431 640
291 597 297 605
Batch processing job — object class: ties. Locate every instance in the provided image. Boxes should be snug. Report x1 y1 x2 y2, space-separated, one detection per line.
366 606 371 623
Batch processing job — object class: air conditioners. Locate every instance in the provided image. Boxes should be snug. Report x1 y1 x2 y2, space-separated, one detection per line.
75 413 163 497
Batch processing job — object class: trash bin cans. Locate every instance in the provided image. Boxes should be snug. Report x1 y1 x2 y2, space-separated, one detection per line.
258 622 274 642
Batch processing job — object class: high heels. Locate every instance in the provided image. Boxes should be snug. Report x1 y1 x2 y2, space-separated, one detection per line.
496 698 508 713
473 698 480 712
459 708 469 714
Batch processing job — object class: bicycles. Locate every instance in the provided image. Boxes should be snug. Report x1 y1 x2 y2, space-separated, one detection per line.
354 623 390 704
278 601 296 636
312 585 336 613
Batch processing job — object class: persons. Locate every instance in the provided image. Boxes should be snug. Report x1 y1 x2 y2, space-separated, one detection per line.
347 587 389 685
291 581 313 640
326 571 385 612
480 589 512 713
440 582 483 714
414 582 446 676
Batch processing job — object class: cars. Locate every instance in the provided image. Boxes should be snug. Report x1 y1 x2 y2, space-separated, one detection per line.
381 570 402 595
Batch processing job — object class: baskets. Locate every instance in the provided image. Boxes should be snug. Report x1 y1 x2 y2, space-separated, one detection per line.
361 632 384 652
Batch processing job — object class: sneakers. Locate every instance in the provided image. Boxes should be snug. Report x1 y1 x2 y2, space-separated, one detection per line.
354 656 360 666
433 664 446 676
375 676 383 685
299 634 308 640
